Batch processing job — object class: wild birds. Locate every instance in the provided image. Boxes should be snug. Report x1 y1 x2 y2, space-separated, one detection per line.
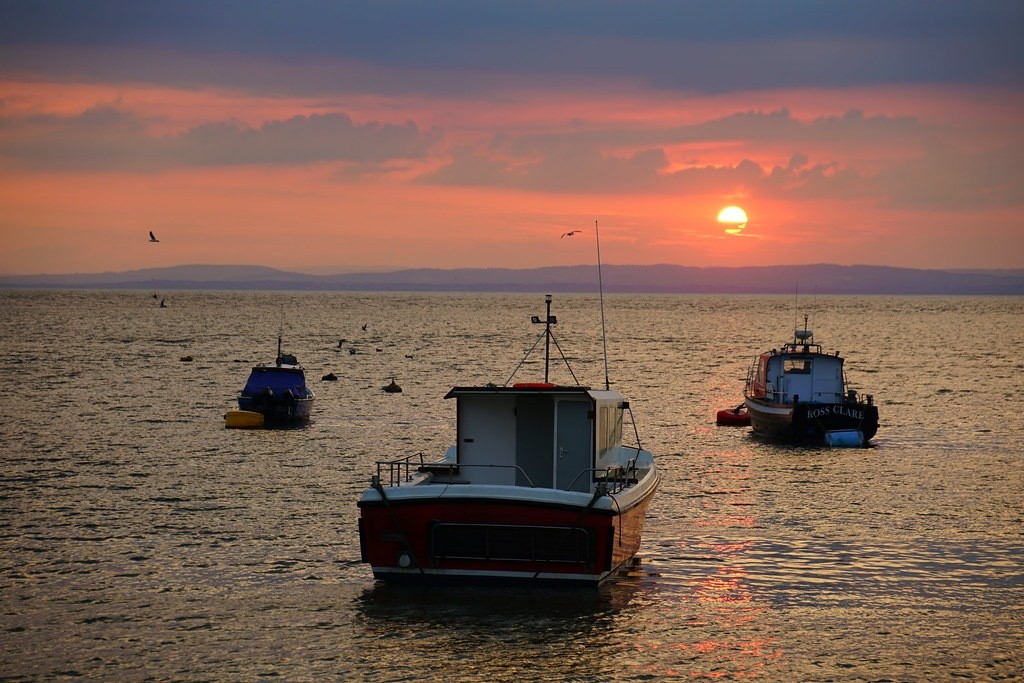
160 299 166 308
561 231 582 240
361 323 368 331
337 339 347 348
148 231 160 242
152 293 157 298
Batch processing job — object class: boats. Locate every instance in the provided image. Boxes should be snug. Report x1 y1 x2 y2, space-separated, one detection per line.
741 277 882 449
357 218 663 595
223 320 318 430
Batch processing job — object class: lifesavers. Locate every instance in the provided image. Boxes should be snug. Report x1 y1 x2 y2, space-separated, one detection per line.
513 382 556 388
